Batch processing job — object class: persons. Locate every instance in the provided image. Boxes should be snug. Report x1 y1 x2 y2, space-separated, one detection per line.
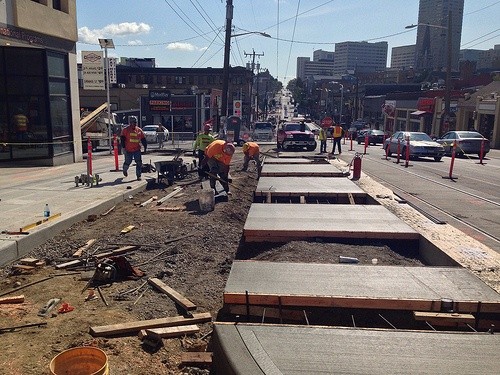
319 124 327 153
193 124 215 174
242 142 262 180
204 140 235 195
121 116 147 181
330 122 344 155
13 108 31 143
156 123 166 149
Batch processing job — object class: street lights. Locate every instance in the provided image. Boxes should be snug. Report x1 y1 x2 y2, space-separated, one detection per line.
330 81 344 126
220 31 271 130
405 24 453 133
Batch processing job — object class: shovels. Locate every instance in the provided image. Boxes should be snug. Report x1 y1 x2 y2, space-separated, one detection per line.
207 173 260 202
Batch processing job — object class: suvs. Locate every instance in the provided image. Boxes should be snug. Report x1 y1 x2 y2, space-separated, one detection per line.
252 122 274 142
276 121 317 151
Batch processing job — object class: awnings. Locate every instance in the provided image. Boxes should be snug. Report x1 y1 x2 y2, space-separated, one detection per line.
410 111 426 117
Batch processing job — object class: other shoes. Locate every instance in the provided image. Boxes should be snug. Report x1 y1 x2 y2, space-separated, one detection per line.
122 168 128 177
137 175 142 181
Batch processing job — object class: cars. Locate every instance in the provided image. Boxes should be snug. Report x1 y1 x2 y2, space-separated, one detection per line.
141 125 169 144
435 131 491 159
326 125 366 140
383 130 446 162
355 129 389 146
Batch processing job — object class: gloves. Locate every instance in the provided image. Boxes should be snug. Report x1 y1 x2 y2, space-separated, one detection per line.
193 150 197 157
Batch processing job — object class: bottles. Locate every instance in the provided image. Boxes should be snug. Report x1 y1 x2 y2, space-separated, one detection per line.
44 204 50 218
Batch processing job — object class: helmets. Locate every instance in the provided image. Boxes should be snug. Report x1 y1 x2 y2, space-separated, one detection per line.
223 143 235 156
204 124 212 130
243 142 250 151
129 116 137 122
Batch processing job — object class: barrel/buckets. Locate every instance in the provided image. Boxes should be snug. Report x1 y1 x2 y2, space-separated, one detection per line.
49 346 110 375
197 188 215 211
